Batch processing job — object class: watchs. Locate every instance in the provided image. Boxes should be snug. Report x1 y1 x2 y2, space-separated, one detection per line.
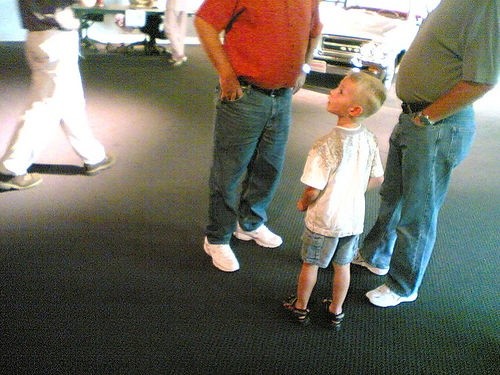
418 111 432 126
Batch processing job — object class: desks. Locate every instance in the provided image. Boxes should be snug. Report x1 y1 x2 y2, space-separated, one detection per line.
73 7 192 56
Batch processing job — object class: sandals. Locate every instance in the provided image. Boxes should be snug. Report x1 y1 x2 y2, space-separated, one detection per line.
281 295 310 322
321 297 345 331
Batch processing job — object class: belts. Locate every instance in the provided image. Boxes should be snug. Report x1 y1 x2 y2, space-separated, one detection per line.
238 77 294 98
401 102 433 114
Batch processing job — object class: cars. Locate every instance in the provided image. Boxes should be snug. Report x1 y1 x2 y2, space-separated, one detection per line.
307 28 396 81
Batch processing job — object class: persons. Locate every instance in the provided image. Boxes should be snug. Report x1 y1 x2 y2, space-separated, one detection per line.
0 0 116 190
164 0 188 65
193 0 324 273
280 72 387 332
348 0 500 308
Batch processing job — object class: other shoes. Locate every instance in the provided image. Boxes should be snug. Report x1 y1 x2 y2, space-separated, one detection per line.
169 56 187 66
84 154 116 176
0 172 42 190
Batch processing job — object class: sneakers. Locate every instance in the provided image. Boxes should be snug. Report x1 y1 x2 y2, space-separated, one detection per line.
351 248 389 275
366 283 417 307
204 235 240 272
232 220 282 248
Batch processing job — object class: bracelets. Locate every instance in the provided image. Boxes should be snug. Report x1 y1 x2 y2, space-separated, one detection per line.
302 64 311 74
